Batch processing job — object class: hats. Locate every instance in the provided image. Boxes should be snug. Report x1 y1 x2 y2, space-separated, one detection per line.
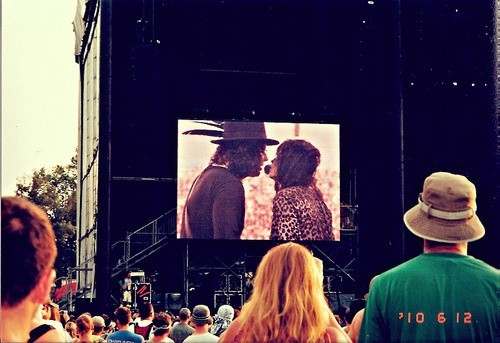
92 316 106 327
190 305 213 323
403 172 485 243
210 120 279 145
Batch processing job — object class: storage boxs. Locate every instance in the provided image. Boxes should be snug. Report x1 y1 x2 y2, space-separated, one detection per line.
325 293 355 313
165 293 182 315
214 275 244 309
124 283 152 311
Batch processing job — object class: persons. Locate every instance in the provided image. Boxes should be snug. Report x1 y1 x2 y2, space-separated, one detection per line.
179 119 280 240
264 139 336 241
119 270 134 309
217 241 354 343
0 196 241 343
357 172 500 343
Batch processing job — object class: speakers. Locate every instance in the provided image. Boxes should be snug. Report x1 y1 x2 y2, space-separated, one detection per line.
75 298 90 319
93 298 108 317
